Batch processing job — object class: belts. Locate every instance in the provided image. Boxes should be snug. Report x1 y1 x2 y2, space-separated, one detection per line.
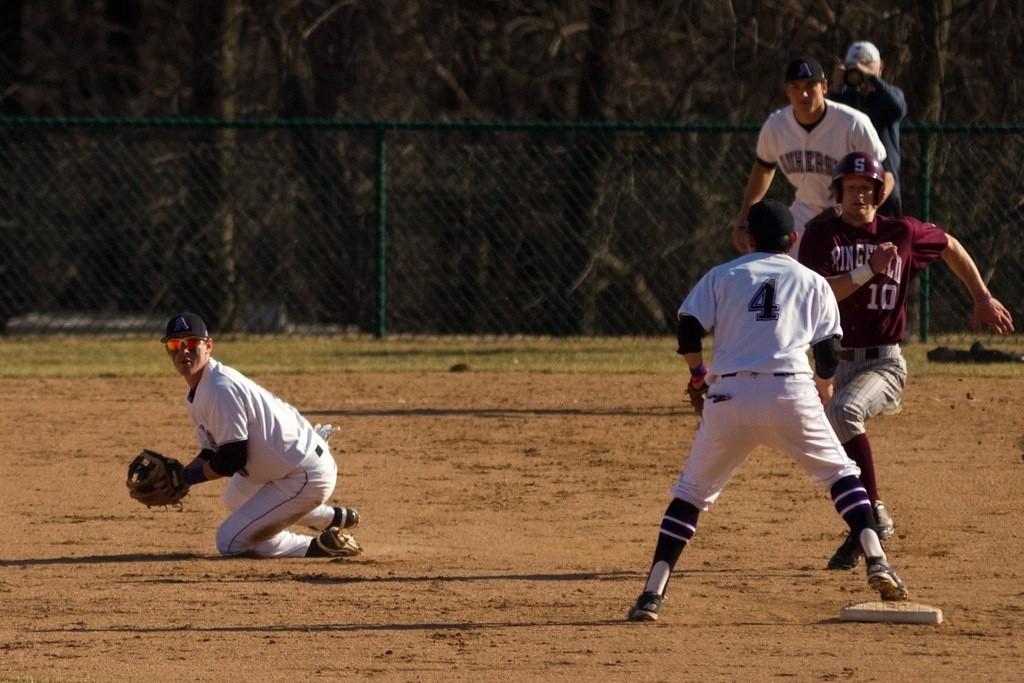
841 349 878 360
721 372 794 379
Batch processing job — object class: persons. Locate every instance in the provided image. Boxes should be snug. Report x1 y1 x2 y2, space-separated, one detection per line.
825 40 907 216
796 151 1015 570
733 56 895 260
627 197 909 622
126 311 364 557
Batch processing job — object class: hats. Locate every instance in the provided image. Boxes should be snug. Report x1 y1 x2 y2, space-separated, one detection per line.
160 313 208 342
745 200 795 238
846 41 882 65
784 57 825 82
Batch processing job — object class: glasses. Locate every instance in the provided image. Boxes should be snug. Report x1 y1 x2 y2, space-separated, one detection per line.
167 337 207 353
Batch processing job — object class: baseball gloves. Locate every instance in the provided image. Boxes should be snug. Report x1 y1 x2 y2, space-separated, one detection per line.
125 448 191 507
685 381 709 415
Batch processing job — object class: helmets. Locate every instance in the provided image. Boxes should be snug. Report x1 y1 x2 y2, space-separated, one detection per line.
831 152 886 204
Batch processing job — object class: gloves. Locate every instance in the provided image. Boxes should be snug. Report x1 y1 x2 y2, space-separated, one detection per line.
705 393 730 403
313 422 341 444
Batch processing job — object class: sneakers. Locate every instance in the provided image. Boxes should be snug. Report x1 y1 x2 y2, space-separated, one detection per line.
827 530 862 571
327 507 359 530
629 591 662 621
866 563 908 601
317 526 362 558
872 505 895 540
